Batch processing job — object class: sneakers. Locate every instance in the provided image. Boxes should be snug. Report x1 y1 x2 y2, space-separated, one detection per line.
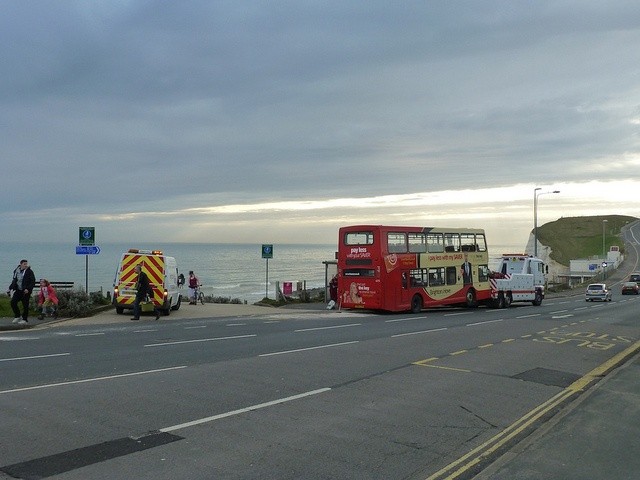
11 318 21 323
130 317 139 320
156 311 160 320
18 320 28 325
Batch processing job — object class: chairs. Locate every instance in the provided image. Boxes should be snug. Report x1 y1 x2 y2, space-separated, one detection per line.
445 246 455 252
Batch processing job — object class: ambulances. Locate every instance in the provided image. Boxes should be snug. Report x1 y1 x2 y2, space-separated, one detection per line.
112 248 185 315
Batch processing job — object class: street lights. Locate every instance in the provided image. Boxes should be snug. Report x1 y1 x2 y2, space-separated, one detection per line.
602 219 609 280
534 187 543 257
536 190 561 204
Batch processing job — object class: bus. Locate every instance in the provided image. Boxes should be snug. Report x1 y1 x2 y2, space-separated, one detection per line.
337 225 492 313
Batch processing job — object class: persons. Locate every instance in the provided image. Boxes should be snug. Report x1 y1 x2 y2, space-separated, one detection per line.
9 260 36 325
459 253 473 288
38 279 59 320
131 266 160 320
189 271 197 305
344 282 363 304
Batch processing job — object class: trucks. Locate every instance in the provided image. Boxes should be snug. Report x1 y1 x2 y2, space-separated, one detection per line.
490 253 548 308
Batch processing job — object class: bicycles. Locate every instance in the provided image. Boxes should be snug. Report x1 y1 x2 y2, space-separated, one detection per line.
189 284 204 306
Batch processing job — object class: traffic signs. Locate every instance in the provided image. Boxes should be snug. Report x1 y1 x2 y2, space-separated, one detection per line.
76 246 100 255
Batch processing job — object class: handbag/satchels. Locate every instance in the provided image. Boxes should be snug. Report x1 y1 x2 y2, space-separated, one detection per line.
141 296 154 312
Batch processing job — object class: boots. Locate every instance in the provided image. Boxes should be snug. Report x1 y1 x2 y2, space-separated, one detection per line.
53 311 59 319
37 313 45 320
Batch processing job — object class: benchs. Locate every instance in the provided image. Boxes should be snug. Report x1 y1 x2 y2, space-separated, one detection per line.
32 282 74 295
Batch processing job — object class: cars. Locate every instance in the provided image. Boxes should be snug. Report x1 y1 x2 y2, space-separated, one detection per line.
629 273 640 285
622 282 640 295
585 283 612 302
